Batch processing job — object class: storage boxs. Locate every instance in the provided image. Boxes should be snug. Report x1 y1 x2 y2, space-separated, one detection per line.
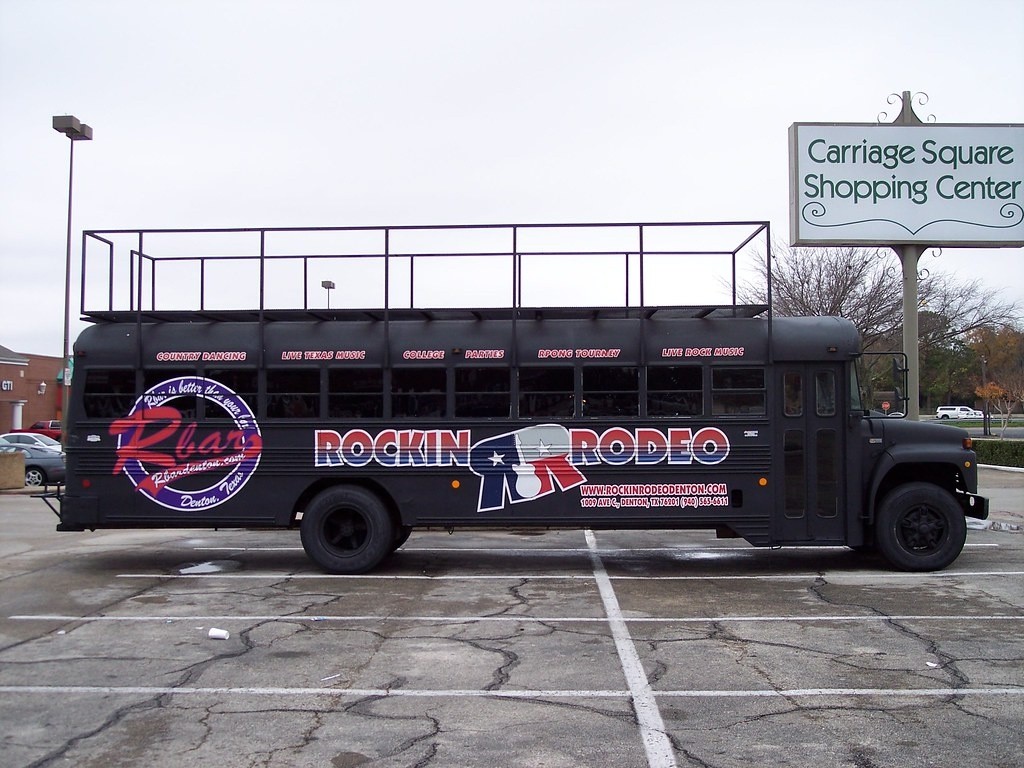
0 450 26 490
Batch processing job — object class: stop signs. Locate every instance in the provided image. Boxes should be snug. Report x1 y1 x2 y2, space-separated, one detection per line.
882 403 891 410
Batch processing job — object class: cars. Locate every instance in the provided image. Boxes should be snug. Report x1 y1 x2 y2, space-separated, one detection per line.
0 432 62 453
7 419 62 445
958 410 994 420
0 444 66 488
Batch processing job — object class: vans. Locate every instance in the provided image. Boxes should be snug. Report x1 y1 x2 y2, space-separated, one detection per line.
937 405 975 421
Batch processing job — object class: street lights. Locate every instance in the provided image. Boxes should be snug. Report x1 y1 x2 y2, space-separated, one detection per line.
54 114 94 454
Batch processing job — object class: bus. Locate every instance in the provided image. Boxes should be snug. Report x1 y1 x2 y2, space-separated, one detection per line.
28 221 991 572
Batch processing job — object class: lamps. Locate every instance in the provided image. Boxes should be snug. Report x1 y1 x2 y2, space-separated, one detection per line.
37 381 47 395
